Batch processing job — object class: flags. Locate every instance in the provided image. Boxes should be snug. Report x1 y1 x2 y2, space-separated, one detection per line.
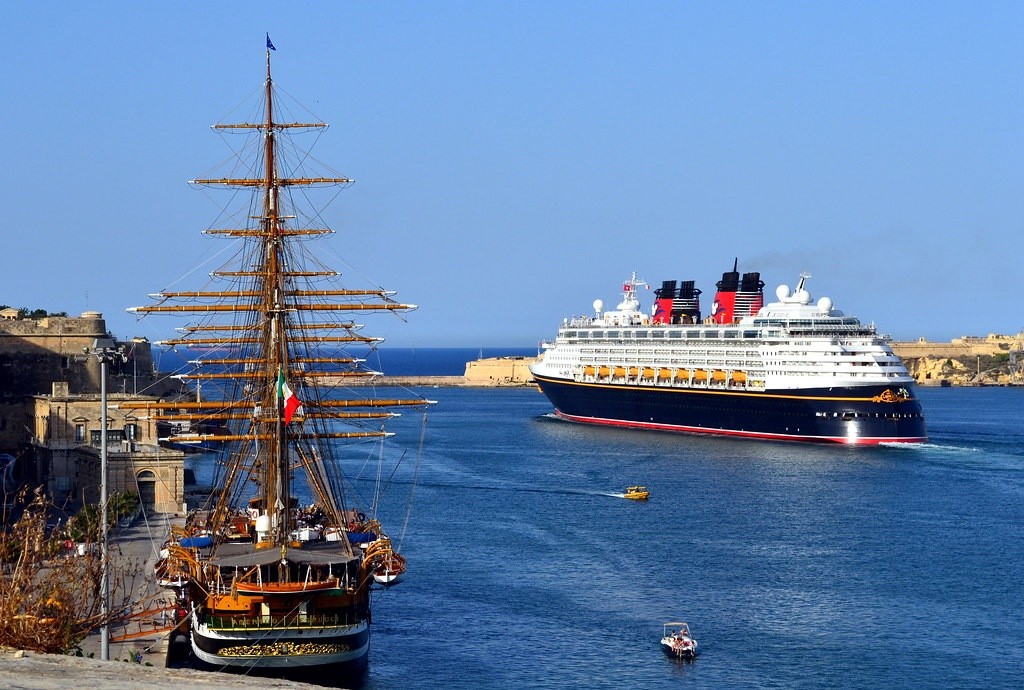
623 284 631 291
276 370 302 429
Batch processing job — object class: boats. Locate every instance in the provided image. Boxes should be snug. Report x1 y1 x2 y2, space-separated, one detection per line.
118 37 439 679
623 483 650 499
658 620 699 661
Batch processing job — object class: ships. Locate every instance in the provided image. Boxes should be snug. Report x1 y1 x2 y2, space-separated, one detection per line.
527 256 930 448
